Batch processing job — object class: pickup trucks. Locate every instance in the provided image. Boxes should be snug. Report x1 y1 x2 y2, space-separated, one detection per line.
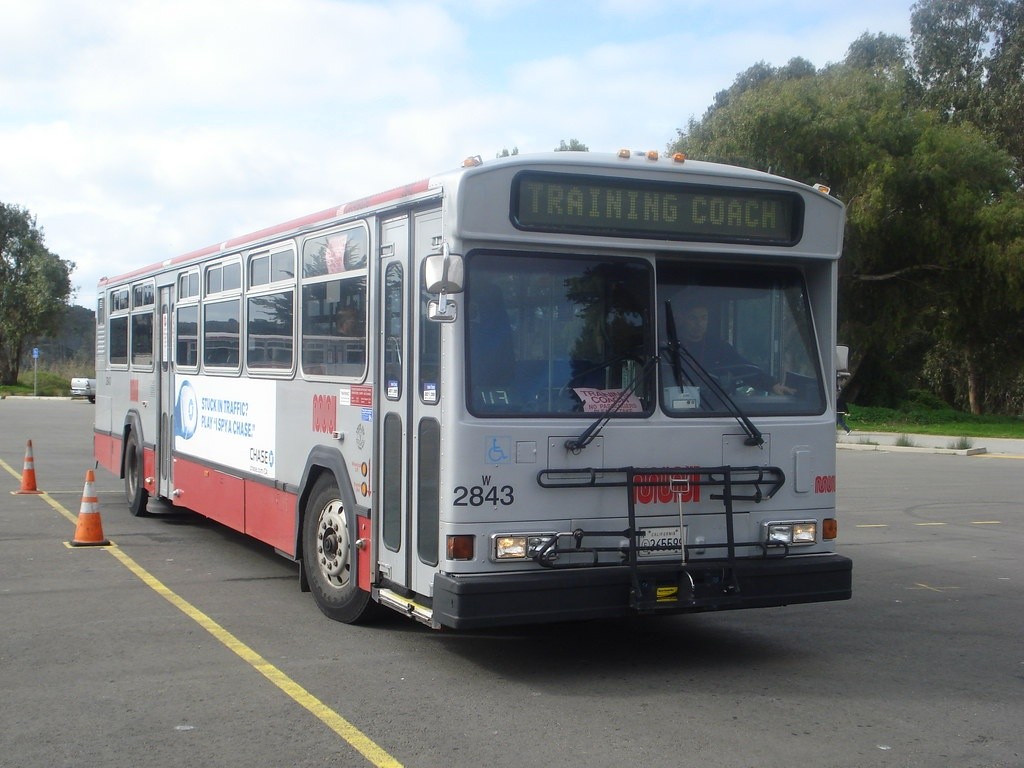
70 377 96 404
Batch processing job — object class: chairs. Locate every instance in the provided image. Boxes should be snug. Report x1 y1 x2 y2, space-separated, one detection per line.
467 286 518 391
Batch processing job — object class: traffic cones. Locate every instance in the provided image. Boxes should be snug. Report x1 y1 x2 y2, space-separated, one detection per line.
17 438 46 494
67 468 113 548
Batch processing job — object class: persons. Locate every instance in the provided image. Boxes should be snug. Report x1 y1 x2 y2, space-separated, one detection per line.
676 304 797 397
837 394 851 436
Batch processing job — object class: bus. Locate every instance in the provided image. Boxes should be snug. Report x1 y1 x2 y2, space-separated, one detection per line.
96 138 857 637
